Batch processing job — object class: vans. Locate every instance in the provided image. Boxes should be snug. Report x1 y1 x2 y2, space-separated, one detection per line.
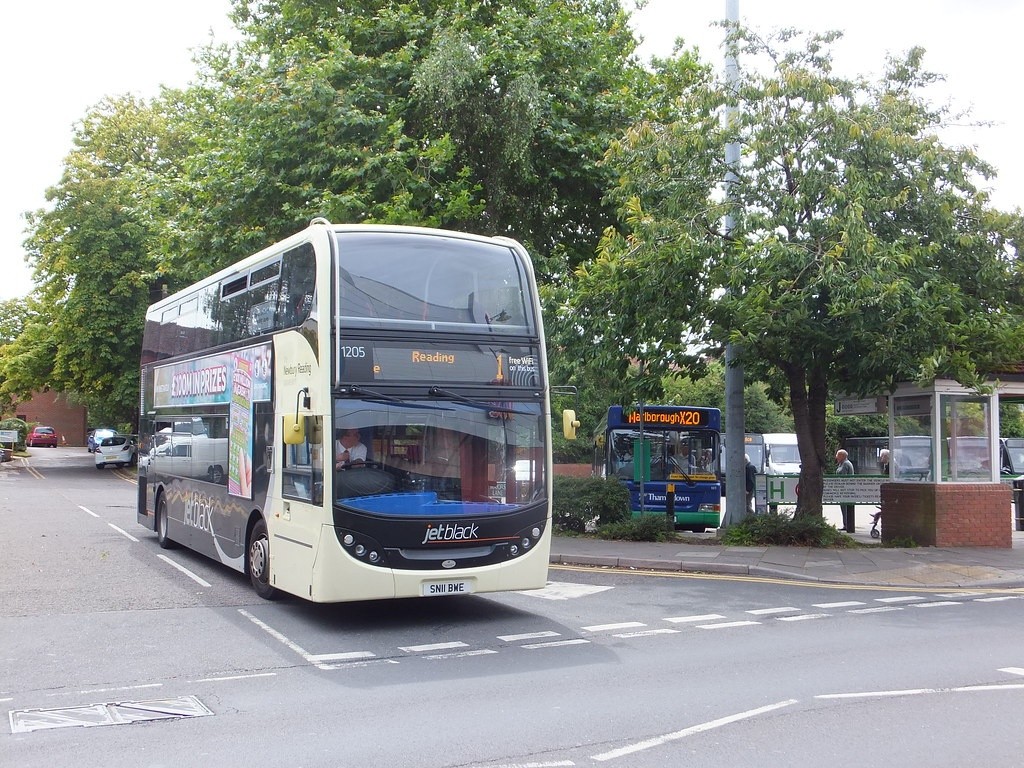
763 432 802 477
139 416 228 484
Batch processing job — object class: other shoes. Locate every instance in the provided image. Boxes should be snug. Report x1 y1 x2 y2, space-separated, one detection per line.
869 521 875 524
870 514 875 518
838 528 846 530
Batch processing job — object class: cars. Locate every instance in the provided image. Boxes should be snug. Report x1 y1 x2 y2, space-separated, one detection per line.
94 434 139 469
25 426 57 449
88 429 119 453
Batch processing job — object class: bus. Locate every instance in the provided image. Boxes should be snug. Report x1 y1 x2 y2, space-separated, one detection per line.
719 432 771 497
844 435 1024 484
136 217 582 603
593 405 721 533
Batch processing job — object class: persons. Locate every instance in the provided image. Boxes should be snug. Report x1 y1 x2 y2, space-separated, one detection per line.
879 449 900 475
239 448 252 497
835 450 855 531
336 423 367 470
745 453 756 514
674 444 696 475
619 442 633 468
700 450 712 470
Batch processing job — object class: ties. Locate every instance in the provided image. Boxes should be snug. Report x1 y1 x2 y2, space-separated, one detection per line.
344 450 351 471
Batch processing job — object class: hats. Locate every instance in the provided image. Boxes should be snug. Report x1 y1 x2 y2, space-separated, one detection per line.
627 445 634 449
681 438 690 445
